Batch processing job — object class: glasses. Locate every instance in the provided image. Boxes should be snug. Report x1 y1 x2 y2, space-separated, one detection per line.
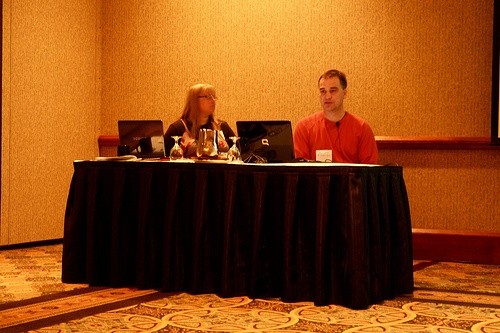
197 94 217 100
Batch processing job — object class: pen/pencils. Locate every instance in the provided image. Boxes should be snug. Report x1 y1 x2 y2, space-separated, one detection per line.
189 156 196 158
298 160 316 162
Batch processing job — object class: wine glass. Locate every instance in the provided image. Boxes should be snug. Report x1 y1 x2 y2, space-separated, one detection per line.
170 135 183 160
227 137 241 161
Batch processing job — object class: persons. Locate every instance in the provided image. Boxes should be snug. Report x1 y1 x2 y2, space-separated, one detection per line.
294 70 378 164
163 84 236 157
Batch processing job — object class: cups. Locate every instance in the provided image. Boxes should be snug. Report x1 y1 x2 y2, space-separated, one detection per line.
118 144 131 156
197 129 217 157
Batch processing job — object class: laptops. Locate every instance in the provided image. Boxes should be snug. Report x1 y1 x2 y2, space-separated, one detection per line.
117 120 172 158
236 120 315 163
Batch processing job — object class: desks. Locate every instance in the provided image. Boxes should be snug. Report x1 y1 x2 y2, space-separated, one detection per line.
62 158 414 310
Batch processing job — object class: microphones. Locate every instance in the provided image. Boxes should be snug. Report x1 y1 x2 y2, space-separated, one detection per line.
336 121 341 126
